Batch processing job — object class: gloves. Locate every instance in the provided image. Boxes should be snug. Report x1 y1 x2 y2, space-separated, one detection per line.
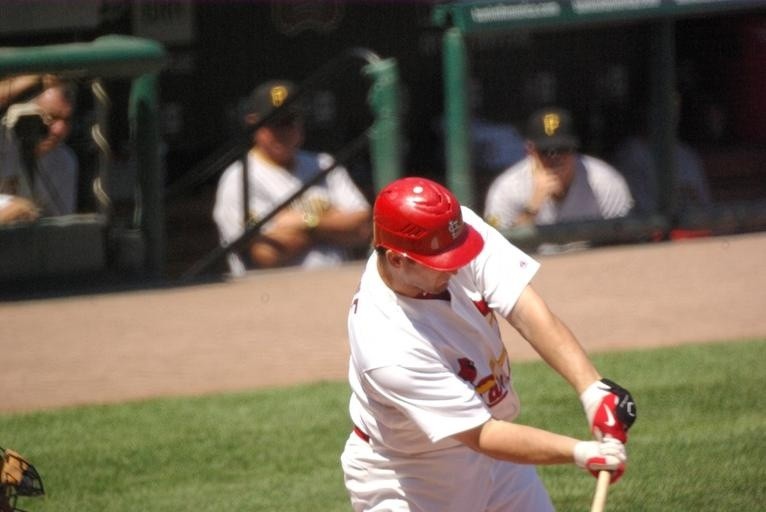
579 375 639 442
574 436 627 484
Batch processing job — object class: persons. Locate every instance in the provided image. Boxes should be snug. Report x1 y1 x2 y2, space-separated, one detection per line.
0 81 77 225
213 78 374 275
336 177 638 510
484 106 635 253
617 112 707 211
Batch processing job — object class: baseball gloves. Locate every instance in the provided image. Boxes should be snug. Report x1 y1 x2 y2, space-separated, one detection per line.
0 447 45 512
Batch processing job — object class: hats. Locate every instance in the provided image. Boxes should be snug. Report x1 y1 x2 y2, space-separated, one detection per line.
525 105 588 151
242 77 309 123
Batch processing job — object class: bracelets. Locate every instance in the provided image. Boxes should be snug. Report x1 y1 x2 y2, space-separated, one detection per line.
523 203 539 215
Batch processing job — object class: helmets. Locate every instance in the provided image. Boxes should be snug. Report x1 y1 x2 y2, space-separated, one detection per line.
371 176 485 272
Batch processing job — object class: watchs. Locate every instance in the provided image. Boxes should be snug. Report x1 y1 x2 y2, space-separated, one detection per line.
304 212 319 231
